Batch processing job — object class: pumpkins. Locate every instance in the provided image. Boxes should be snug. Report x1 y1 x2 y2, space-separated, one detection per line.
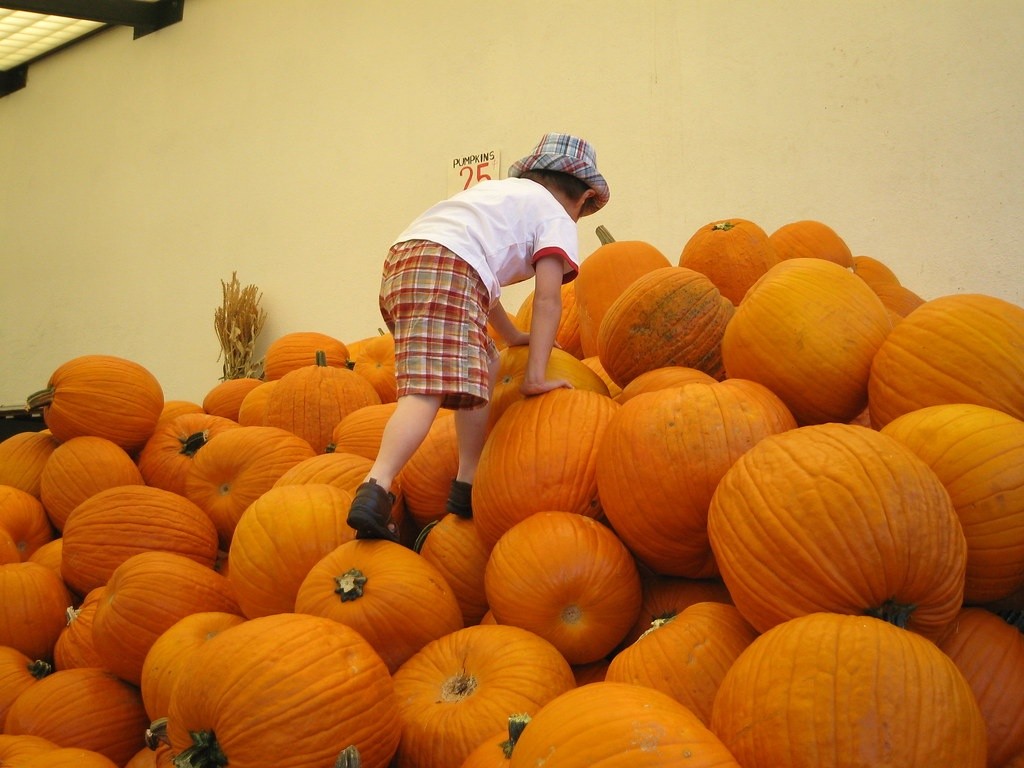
0 218 1024 768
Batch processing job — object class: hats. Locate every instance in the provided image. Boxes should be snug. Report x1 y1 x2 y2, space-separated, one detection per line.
507 132 610 217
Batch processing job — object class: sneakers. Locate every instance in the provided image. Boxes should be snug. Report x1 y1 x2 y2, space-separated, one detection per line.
445 478 473 517
346 478 401 545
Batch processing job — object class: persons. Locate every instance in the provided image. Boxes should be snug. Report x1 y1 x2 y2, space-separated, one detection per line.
346 133 609 541
